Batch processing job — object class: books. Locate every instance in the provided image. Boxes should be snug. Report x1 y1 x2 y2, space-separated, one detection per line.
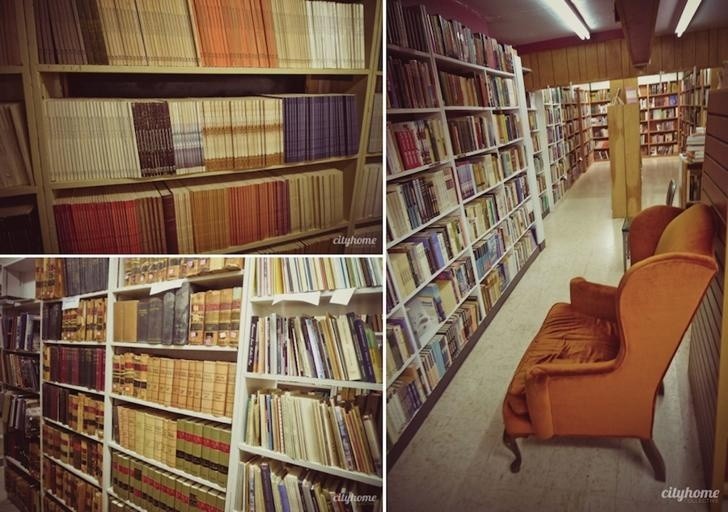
591 66 712 163
1 2 590 510
687 167 703 203
